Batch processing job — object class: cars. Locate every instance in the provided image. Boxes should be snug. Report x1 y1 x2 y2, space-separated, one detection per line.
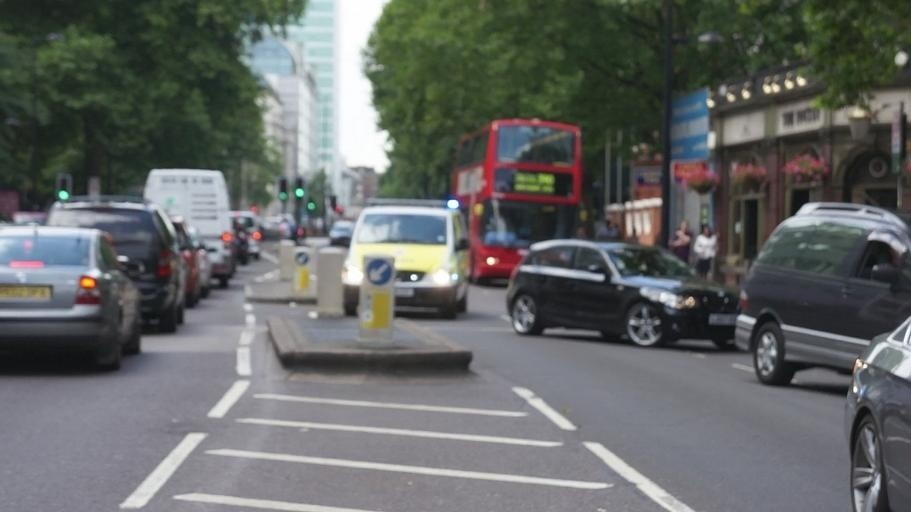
330 220 353 245
505 240 739 353
133 166 303 301
835 310 911 512
0 222 141 372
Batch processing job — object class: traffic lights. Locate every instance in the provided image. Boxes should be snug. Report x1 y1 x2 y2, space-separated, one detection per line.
307 198 314 216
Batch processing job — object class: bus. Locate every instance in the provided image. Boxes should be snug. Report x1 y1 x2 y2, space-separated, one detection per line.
451 119 581 288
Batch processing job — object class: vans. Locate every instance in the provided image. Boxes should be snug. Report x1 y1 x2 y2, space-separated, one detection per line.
722 198 911 387
43 199 188 334
337 200 470 314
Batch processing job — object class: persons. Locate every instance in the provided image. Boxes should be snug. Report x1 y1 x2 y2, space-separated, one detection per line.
693 223 719 279
667 219 693 263
595 219 620 239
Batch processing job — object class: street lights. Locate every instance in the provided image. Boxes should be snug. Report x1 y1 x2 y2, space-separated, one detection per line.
658 26 727 245
